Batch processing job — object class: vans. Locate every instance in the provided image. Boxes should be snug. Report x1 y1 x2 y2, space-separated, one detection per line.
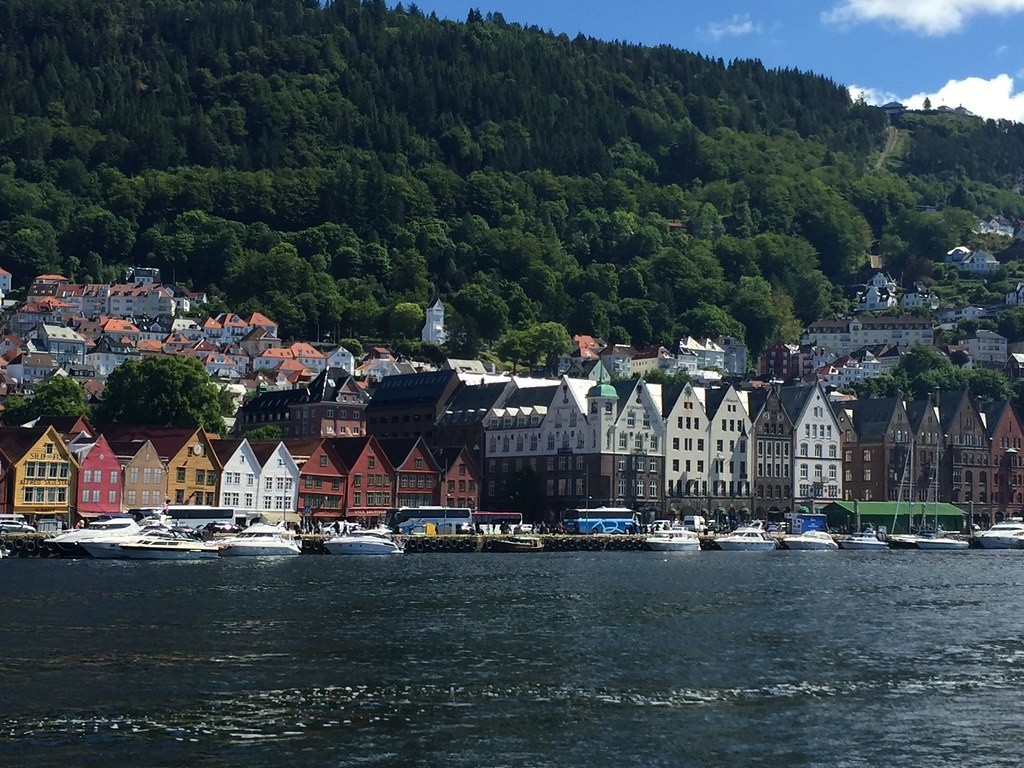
684 516 701 533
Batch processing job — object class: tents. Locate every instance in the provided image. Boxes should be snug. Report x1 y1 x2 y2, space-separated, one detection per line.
820 501 971 534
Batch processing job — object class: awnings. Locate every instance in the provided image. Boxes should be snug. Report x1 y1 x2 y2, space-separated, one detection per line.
699 507 752 516
668 507 677 515
78 512 105 517
797 506 825 513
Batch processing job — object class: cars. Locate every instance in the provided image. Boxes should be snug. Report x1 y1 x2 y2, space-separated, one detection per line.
651 520 671 531
521 524 534 534
0 520 35 535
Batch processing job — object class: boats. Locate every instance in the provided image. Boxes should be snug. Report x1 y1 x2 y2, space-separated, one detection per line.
322 528 405 555
836 531 890 550
118 529 222 561
888 434 1024 551
79 509 185 559
782 530 839 551
714 526 779 551
42 513 143 555
644 529 701 550
491 537 545 552
215 522 303 555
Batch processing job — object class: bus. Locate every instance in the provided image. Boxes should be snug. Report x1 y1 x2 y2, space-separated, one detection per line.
559 507 638 535
469 511 524 536
388 505 473 536
127 504 236 533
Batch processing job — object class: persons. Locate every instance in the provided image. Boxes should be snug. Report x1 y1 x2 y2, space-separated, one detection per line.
629 522 670 535
435 522 439 534
286 519 389 537
76 519 85 529
18 519 39 531
829 523 871 534
708 520 782 534
63 523 67 530
468 519 566 535
